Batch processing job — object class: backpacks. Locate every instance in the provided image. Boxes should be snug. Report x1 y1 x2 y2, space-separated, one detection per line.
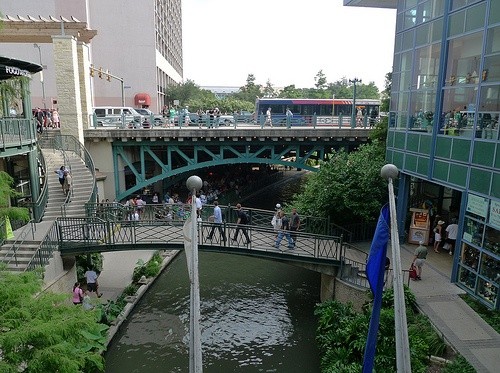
246 214 250 223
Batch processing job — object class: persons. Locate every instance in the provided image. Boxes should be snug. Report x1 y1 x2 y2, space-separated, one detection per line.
432 221 445 254
356 106 364 128
128 117 138 130
370 107 379 128
142 117 151 130
54 166 75 204
85 265 103 298
73 283 84 306
284 105 294 129
270 202 302 250
28 105 61 135
160 102 240 130
262 107 275 129
389 103 500 140
231 202 252 244
196 195 205 218
206 199 228 243
99 158 296 222
414 241 429 280
445 219 459 256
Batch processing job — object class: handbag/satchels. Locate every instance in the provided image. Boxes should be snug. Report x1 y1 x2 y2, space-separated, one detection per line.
433 228 437 233
79 293 84 302
409 261 417 278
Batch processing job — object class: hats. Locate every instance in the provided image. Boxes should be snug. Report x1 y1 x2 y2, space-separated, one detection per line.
438 220 445 224
212 201 219 204
451 218 458 223
436 215 441 217
418 241 425 244
235 204 242 207
87 264 94 270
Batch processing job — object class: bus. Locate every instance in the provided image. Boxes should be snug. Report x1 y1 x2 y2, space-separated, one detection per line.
254 98 382 127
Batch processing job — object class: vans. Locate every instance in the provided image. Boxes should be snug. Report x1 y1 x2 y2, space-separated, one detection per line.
21 108 60 127
93 105 144 127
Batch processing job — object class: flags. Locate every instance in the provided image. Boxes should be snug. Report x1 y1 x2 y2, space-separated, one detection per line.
361 196 398 373
182 218 213 373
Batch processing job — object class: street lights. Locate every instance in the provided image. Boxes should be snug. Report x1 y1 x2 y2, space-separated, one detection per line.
348 78 362 128
33 43 47 108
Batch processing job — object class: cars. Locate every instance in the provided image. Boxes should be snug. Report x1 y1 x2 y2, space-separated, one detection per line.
213 115 236 128
233 111 253 124
136 108 163 127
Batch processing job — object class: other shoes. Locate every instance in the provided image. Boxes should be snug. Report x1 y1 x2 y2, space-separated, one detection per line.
232 238 237 241
98 292 103 298
243 240 251 244
220 238 227 241
206 237 212 239
273 245 280 248
415 277 421 280
435 251 440 254
288 245 294 248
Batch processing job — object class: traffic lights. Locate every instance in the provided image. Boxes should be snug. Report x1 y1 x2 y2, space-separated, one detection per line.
90 64 95 77
98 66 103 79
106 70 111 82
